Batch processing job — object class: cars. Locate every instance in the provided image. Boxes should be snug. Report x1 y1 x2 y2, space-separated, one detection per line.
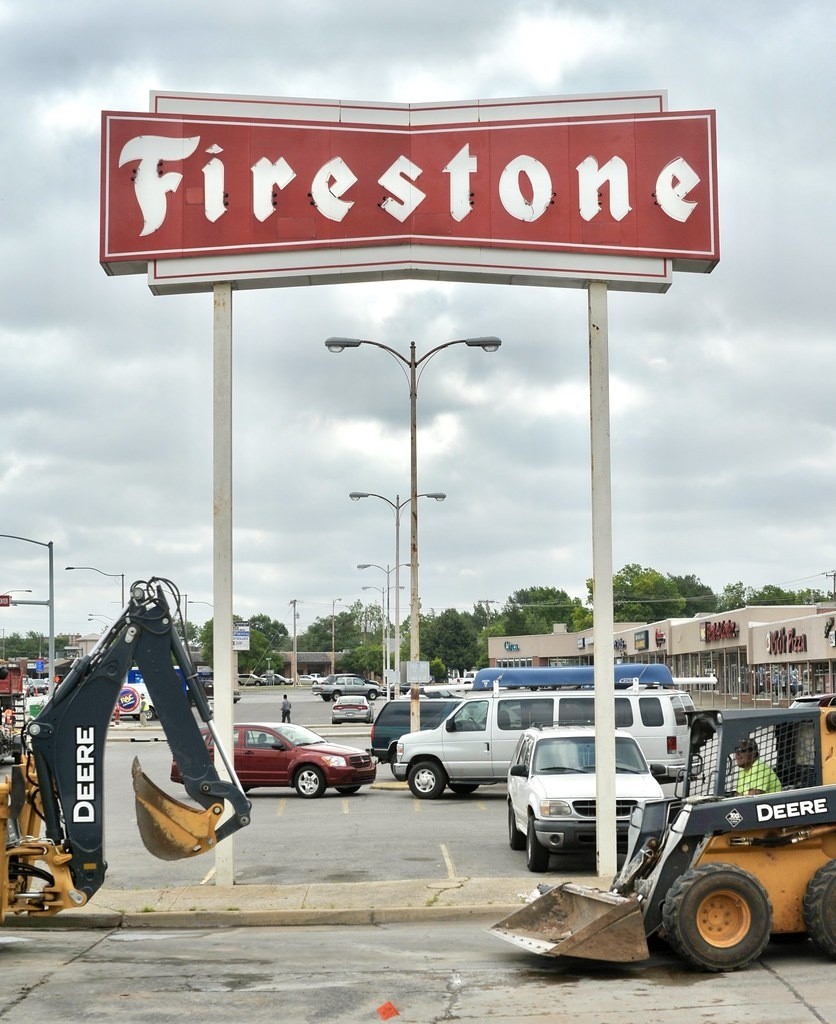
171 722 376 798
260 674 293 685
198 679 241 703
298 674 319 685
22 674 63 697
788 692 836 708
405 684 526 695
238 673 268 686
331 696 374 724
382 679 427 698
367 698 465 781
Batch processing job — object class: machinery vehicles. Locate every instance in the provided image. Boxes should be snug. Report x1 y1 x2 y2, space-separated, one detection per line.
0 577 252 926
485 711 835 980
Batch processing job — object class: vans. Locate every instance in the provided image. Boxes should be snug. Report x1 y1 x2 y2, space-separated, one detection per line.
393 663 695 799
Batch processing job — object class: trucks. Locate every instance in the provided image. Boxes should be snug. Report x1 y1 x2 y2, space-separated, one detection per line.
0 658 27 764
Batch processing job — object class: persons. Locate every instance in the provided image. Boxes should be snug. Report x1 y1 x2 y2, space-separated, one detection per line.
280 694 292 723
734 739 783 795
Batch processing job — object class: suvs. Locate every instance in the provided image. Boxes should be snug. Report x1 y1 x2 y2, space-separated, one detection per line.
506 722 665 872
321 673 379 685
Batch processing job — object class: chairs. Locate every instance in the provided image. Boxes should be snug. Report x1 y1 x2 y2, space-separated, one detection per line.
258 734 271 747
248 733 257 747
498 710 511 730
539 753 563 769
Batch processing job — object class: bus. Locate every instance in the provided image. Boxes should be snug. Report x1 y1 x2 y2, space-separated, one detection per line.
111 665 189 720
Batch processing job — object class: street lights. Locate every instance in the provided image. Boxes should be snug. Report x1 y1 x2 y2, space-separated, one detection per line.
324 332 503 733
1 565 213 652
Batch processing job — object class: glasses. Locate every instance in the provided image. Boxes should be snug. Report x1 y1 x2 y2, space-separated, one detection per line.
735 747 745 753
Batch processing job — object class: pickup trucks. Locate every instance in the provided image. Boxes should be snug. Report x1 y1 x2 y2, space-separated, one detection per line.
311 677 381 701
310 673 327 684
455 671 479 684
